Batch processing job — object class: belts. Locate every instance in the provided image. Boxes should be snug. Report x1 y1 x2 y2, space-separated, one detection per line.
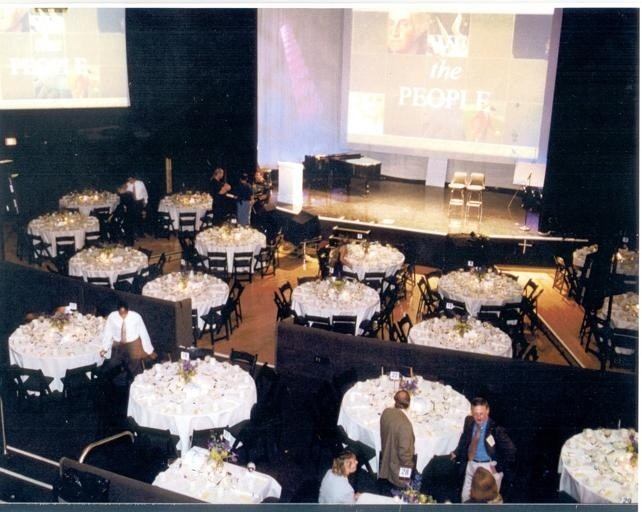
473 459 490 463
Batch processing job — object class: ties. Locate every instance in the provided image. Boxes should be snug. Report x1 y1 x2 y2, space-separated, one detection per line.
467 424 482 463
132 185 136 200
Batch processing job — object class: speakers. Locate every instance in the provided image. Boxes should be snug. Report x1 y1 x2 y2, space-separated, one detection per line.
271 169 278 186
283 211 321 246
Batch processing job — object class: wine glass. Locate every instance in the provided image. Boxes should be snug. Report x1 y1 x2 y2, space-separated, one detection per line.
355 371 451 432
166 449 248 500
16 313 105 356
586 430 638 489
137 360 250 413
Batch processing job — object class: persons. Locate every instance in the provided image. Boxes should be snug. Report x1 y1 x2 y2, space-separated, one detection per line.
206 165 234 234
377 389 416 488
383 12 430 61
317 238 333 282
331 239 352 282
248 169 273 233
109 182 141 250
123 170 150 241
233 173 254 227
318 449 360 504
461 465 503 503
449 396 517 502
100 303 160 390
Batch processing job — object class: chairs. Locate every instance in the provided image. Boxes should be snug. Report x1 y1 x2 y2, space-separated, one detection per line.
0 188 637 505
464 172 488 204
444 170 467 199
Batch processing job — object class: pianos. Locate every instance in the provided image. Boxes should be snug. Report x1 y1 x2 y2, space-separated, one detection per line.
332 155 382 197
